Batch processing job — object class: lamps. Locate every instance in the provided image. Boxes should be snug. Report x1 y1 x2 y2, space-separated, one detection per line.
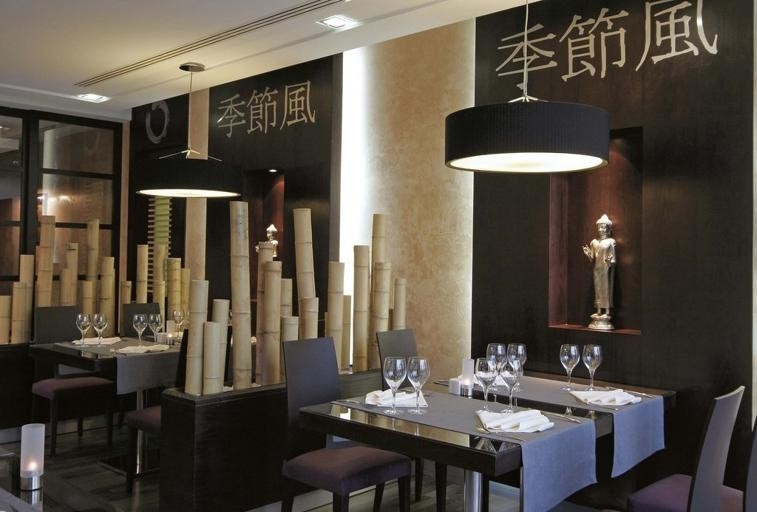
443 0 611 177
133 61 244 199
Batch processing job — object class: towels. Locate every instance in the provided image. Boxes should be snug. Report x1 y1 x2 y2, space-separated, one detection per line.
572 388 642 407
454 369 508 387
362 386 429 409
72 336 120 346
474 407 556 432
119 344 170 354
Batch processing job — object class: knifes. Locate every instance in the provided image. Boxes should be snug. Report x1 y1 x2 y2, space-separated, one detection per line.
336 399 359 404
608 386 652 398
588 401 618 411
541 410 580 424
400 388 429 396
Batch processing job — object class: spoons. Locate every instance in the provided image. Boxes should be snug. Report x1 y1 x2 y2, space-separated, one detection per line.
475 425 525 442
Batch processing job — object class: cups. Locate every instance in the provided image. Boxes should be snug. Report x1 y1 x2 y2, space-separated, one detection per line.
166 319 174 345
459 357 474 396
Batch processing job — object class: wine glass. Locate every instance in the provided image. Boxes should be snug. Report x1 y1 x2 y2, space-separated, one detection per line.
581 344 604 392
170 309 183 339
475 342 526 414
406 356 430 414
91 313 108 347
148 314 162 347
76 313 89 349
559 343 579 392
382 356 406 417
133 313 148 348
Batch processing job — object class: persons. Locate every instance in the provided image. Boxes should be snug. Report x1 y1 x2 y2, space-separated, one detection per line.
582 213 616 317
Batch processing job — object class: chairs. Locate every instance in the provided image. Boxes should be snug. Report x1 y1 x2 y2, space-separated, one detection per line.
121 325 236 500
627 411 756 512
686 384 746 512
27 305 115 457
279 337 412 512
117 300 173 428
376 330 489 511
